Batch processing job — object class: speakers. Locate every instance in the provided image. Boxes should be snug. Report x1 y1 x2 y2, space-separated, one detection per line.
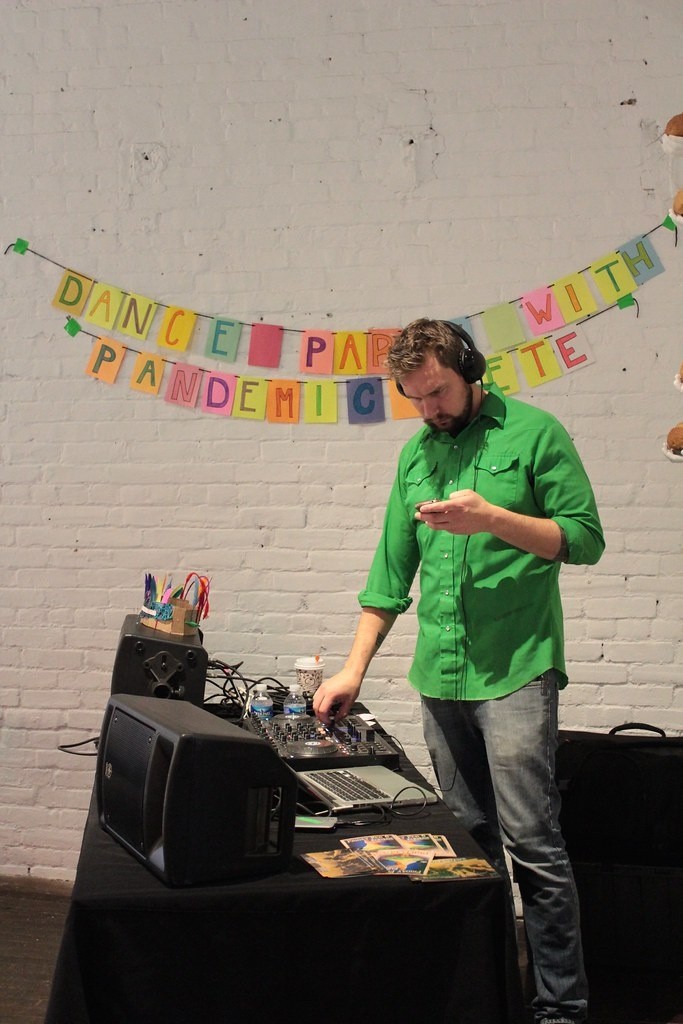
96 694 299 888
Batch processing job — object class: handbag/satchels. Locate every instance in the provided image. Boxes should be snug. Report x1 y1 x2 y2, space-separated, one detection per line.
555 723 682 868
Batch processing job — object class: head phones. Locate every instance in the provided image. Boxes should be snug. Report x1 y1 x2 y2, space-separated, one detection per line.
396 320 486 395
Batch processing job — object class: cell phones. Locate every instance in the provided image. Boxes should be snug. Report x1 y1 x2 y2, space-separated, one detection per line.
415 499 440 512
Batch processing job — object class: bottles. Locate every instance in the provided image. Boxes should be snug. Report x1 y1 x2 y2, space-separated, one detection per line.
283 685 306 715
251 684 273 720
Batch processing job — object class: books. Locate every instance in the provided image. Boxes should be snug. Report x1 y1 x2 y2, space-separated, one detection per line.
299 832 501 883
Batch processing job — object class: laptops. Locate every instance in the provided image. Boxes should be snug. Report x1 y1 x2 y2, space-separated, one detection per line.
280 759 438 813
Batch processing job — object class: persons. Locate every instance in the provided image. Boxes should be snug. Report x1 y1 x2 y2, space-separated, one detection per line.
312 315 607 1023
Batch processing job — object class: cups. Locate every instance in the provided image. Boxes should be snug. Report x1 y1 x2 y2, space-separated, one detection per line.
294 658 325 696
